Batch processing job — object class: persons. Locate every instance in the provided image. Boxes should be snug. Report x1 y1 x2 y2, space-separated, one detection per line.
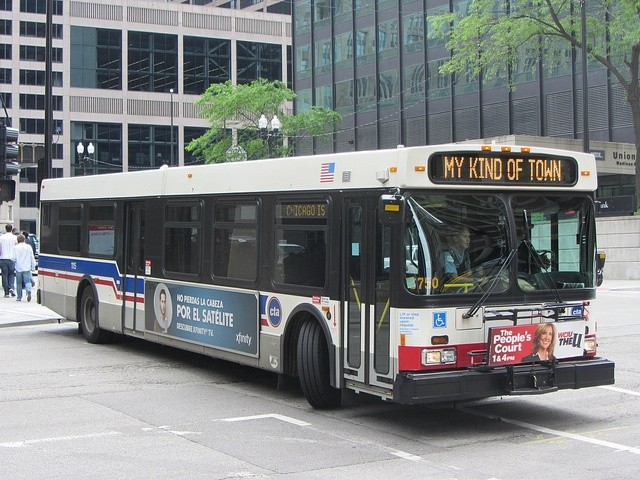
516 226 550 274
0 224 18 298
435 225 471 292
521 322 556 362
10 235 36 302
12 228 19 236
153 289 172 335
22 232 36 289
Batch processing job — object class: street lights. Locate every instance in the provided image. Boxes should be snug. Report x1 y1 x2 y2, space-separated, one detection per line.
76 141 95 175
258 114 281 159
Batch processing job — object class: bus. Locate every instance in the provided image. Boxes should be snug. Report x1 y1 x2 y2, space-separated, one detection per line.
37 141 615 410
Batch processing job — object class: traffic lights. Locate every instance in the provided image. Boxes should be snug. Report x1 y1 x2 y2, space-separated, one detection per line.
0 179 16 201
0 124 19 178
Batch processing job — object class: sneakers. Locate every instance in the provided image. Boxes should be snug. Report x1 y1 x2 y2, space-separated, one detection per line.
32 281 35 287
4 295 9 298
9 289 16 297
16 299 21 301
27 291 31 302
